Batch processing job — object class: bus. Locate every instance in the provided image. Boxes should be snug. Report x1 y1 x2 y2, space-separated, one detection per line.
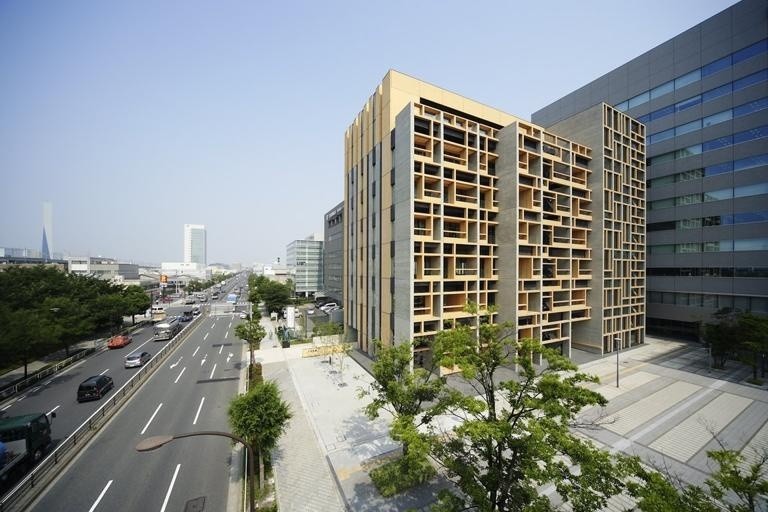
226 287 241 304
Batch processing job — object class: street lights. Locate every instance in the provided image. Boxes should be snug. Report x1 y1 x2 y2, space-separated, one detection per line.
615 337 621 388
135 430 256 512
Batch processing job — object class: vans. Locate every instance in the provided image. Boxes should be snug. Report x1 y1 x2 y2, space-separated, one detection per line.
152 307 164 314
182 308 200 321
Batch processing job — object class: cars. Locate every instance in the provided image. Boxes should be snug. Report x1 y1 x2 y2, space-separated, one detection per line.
108 336 132 349
77 376 113 403
125 352 150 368
186 292 219 303
239 311 247 319
307 308 314 315
316 301 339 314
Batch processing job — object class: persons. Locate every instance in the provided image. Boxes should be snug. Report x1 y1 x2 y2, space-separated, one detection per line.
269 330 272 340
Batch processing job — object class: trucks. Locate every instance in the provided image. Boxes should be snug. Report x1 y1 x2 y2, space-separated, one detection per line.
1 413 57 486
153 318 183 341
